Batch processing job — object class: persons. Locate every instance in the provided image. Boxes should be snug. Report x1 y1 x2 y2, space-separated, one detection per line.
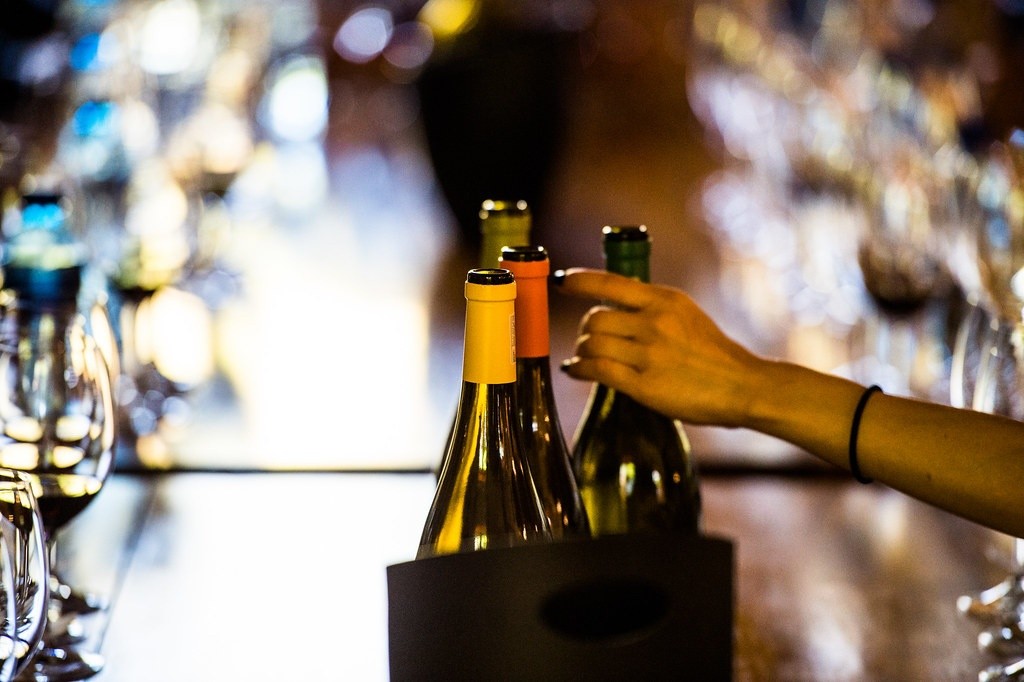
553 268 1024 541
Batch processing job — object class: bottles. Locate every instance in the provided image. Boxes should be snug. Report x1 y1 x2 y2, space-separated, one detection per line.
499 246 594 540
572 222 699 541
479 199 532 267
415 269 553 566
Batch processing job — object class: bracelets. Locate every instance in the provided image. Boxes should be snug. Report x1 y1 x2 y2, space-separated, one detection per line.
848 384 883 484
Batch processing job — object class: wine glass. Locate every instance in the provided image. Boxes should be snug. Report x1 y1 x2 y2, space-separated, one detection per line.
0 301 117 682
905 303 1024 682
